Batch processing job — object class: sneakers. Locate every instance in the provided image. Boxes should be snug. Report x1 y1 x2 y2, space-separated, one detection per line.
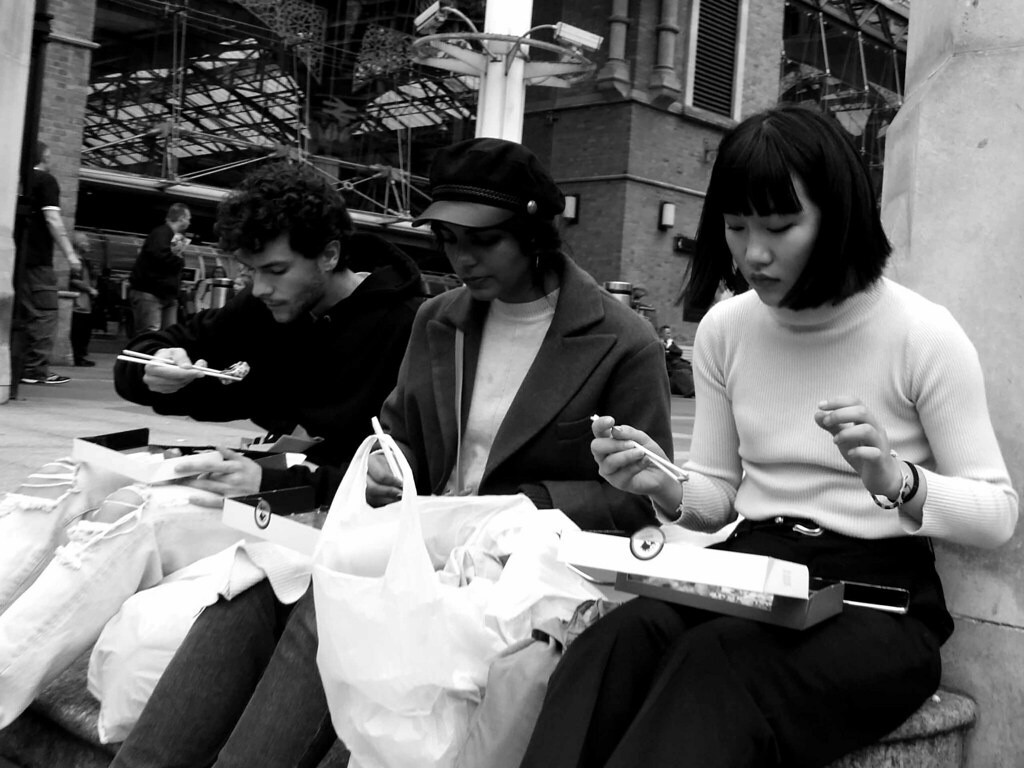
21 370 72 384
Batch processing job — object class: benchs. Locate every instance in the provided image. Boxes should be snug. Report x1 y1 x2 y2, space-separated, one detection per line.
830 688 977 768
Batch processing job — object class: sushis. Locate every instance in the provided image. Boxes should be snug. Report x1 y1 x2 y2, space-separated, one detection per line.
219 361 250 385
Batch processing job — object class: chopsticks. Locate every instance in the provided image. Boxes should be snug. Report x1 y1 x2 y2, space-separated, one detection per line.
116 349 243 381
590 413 702 484
371 414 404 489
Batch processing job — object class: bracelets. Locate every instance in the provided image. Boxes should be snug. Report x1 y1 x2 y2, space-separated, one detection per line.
870 450 920 510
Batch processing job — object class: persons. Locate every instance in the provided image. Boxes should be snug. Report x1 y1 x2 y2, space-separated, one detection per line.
106 135 676 768
660 324 696 398
517 109 1020 768
12 140 83 386
68 228 98 367
128 201 194 344
1 156 428 742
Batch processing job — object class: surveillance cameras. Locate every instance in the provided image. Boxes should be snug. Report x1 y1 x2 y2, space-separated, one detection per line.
414 0 454 34
553 22 605 53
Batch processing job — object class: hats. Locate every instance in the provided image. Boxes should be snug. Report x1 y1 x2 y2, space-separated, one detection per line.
410 137 566 230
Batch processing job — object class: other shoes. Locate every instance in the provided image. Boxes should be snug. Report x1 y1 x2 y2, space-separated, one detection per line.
75 358 95 367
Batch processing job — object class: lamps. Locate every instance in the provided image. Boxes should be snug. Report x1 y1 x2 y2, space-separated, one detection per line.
657 201 676 232
562 194 580 224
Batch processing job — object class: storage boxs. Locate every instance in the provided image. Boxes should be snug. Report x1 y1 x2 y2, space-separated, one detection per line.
560 530 845 632
222 483 377 559
74 427 307 484
